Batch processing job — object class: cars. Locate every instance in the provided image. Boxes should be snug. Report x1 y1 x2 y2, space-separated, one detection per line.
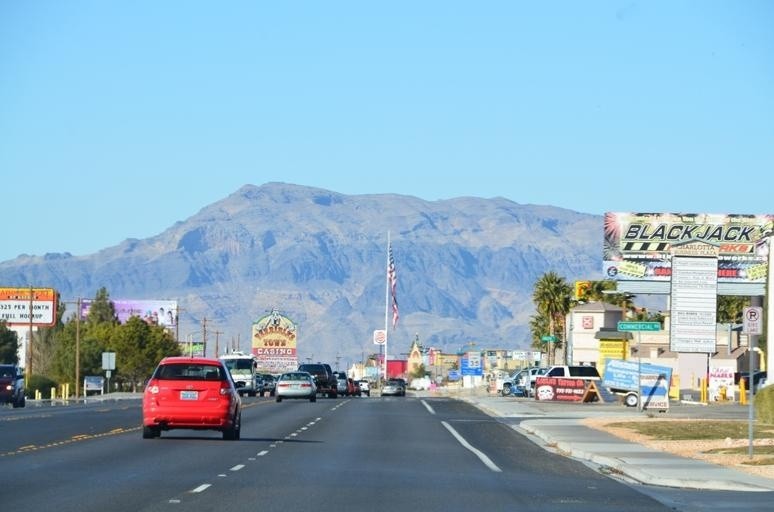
138 355 246 442
733 368 768 396
255 363 408 403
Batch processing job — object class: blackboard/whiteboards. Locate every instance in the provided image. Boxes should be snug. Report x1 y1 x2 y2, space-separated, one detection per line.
592 380 613 403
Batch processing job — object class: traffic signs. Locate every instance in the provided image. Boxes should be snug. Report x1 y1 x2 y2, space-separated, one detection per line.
540 335 557 342
468 351 482 369
615 320 662 333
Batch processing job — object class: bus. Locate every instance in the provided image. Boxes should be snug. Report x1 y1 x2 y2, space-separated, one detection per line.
218 350 258 398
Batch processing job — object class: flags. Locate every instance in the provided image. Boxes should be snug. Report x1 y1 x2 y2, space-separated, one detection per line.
388 244 398 329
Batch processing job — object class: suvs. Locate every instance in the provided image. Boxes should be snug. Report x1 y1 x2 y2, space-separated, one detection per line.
492 365 603 398
0 362 27 408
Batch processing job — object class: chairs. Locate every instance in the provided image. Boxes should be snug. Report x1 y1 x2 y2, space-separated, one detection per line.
206 372 217 379
